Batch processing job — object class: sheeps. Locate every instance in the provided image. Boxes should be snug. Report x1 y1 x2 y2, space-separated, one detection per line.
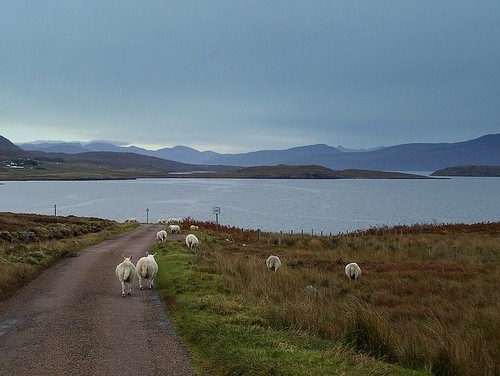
115 254 136 297
136 251 159 290
157 217 188 224
168 225 181 234
156 230 167 245
185 234 198 251
128 218 139 223
344 262 362 281
266 256 281 273
190 225 199 230
